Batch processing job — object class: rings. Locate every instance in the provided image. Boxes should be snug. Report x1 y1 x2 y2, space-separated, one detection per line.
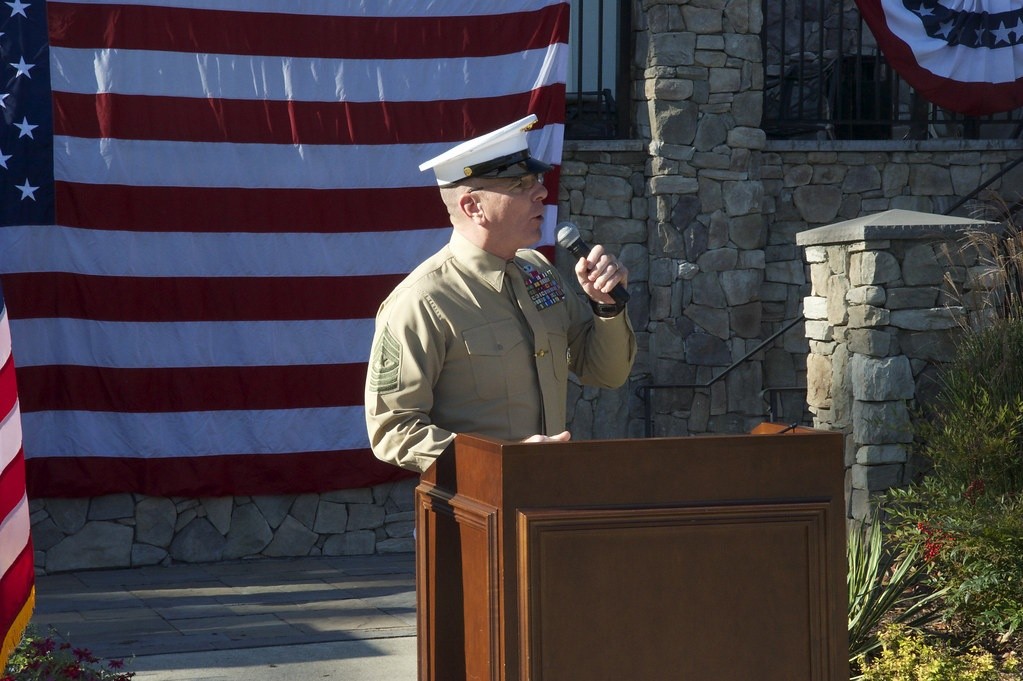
612 262 618 270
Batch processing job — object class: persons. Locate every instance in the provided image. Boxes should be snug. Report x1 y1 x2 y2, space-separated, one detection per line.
363 113 638 539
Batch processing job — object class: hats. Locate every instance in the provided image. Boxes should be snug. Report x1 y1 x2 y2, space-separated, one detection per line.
418 113 553 189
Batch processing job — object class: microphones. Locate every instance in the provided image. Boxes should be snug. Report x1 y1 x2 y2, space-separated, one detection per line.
554 222 631 308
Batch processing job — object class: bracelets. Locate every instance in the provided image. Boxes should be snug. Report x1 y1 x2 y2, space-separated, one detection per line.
588 297 625 315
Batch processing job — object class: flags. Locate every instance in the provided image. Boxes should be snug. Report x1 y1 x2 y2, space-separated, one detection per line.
1 0 571 501
0 296 38 676
